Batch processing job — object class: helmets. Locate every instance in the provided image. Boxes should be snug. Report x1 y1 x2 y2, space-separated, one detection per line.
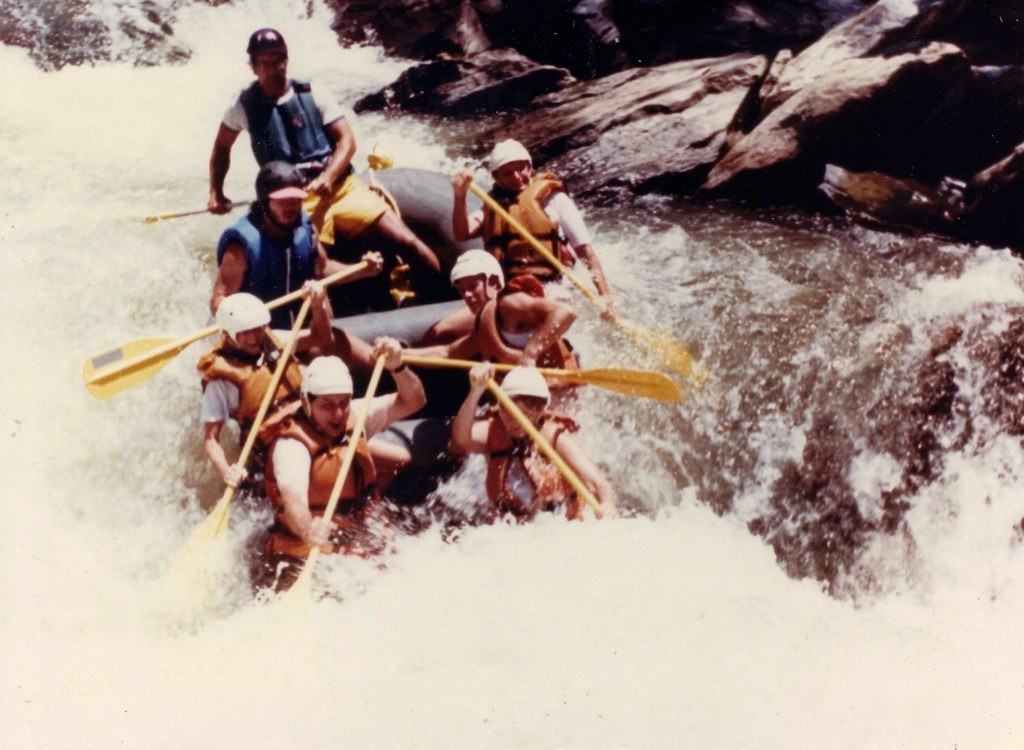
449 249 512 290
300 354 356 416
485 137 533 179
496 364 553 404
214 290 281 343
244 29 288 54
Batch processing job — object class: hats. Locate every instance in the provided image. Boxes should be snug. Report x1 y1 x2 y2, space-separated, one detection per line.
267 186 311 200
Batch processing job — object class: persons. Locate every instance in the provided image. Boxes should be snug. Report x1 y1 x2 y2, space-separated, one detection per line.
196 28 618 595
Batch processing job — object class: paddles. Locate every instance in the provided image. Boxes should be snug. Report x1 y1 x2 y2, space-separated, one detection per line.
486 378 599 511
287 354 387 599
186 294 311 550
145 198 257 224
470 183 707 383
401 354 680 403
82 259 369 400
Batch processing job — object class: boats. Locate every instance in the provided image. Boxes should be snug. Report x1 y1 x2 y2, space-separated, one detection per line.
252 420 639 597
194 162 503 399
